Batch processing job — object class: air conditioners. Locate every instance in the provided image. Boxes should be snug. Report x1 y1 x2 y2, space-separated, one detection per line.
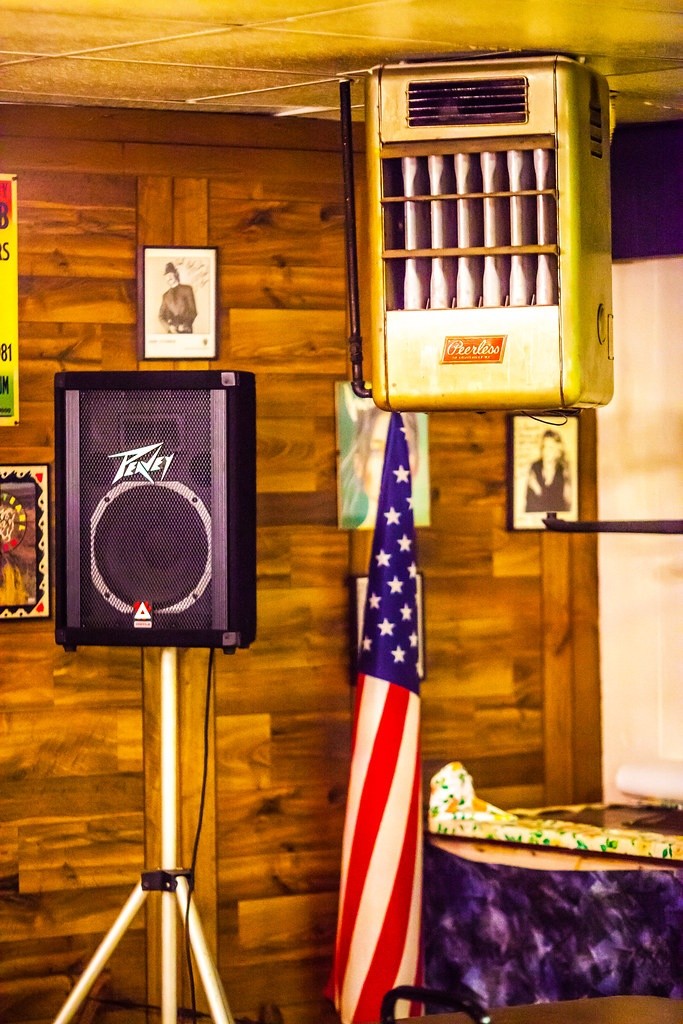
362 65 618 408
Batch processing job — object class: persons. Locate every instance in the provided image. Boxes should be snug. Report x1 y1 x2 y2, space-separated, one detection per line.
159 263 198 333
526 430 570 512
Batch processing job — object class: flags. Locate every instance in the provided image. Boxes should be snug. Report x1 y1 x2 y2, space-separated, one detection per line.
322 413 426 1024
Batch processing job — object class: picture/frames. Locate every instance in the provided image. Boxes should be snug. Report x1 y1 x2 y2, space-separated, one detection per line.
0 463 52 622
506 412 580 532
334 380 432 531
143 246 218 361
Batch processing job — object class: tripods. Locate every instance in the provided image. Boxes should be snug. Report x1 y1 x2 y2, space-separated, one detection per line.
52 646 236 1024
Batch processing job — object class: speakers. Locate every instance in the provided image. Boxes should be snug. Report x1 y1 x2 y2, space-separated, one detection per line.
54 369 258 654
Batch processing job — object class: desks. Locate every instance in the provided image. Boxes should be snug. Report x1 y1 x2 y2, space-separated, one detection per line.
421 803 683 1014
